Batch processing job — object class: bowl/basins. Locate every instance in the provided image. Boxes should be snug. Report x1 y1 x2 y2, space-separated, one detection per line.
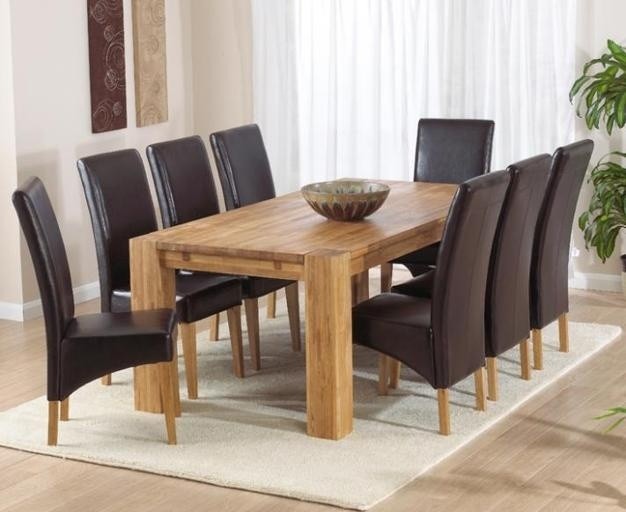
301 181 390 220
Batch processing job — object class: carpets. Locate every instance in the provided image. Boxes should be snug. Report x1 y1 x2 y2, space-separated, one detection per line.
0 294 622 511
